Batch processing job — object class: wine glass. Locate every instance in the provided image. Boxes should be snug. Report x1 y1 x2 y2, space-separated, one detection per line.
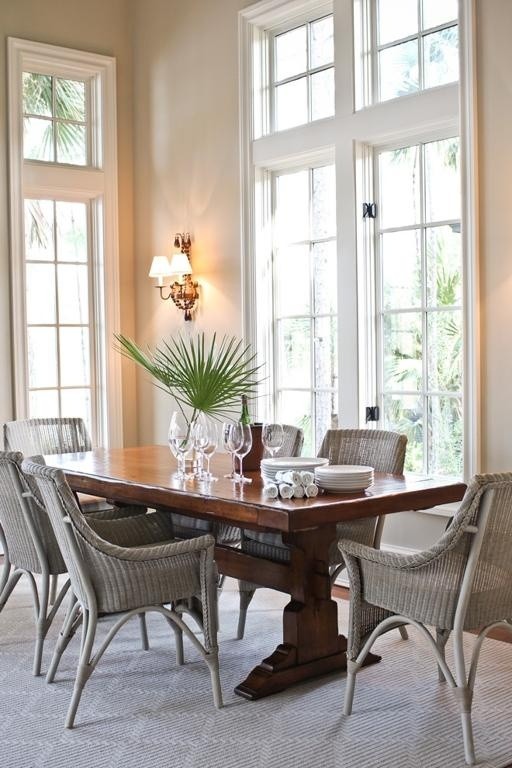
228 424 253 485
196 422 219 483
167 412 196 481
189 420 210 482
222 421 244 479
262 421 284 458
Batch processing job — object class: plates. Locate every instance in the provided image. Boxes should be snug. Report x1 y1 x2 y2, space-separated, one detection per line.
260 456 374 494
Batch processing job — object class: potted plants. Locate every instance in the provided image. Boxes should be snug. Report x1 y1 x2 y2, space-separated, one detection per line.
112 332 269 468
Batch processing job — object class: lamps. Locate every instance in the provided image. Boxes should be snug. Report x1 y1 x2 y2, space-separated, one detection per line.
149 233 199 321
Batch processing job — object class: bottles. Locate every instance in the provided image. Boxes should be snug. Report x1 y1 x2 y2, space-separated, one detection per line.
237 394 253 426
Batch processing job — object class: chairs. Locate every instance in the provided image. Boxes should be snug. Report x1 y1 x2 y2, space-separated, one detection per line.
238 424 408 641
0 417 224 730
337 472 512 766
